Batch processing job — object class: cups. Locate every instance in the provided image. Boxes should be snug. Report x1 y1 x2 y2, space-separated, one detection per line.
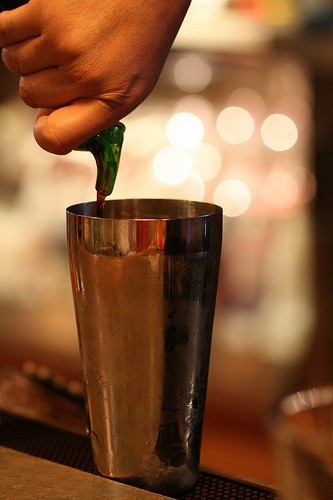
65 199 224 499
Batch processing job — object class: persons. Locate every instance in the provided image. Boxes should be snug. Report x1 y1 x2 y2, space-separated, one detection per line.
0 0 192 156
